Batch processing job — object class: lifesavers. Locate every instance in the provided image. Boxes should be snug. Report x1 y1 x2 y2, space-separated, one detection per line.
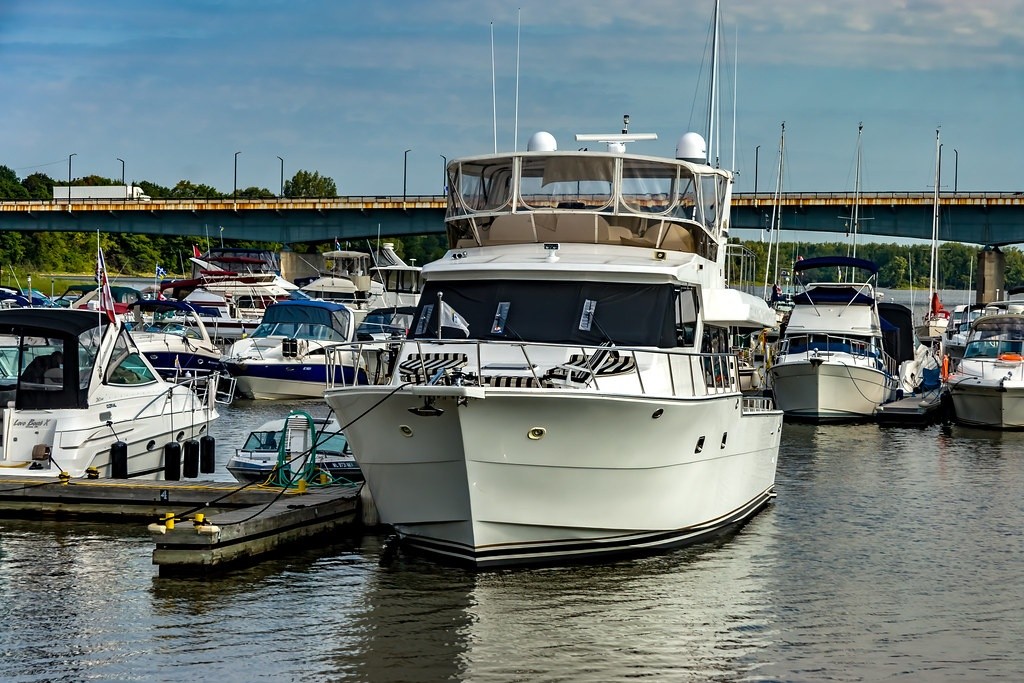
941 353 948 381
1000 353 1023 361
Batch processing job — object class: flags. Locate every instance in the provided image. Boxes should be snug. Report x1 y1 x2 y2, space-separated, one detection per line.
102 271 116 325
157 292 167 301
336 240 340 250
157 266 166 276
440 301 470 337
193 246 202 257
94 250 103 283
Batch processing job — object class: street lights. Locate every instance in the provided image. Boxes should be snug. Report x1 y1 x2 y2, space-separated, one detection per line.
277 156 284 199
68 153 77 203
404 149 412 201
755 145 760 192
939 143 943 191
439 154 446 198
234 152 242 199
953 150 958 194
117 158 125 185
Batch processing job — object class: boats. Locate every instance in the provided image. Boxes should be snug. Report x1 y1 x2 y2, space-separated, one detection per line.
729 122 947 418
139 225 424 401
0 229 238 479
225 408 365 488
909 123 1024 431
306 0 784 561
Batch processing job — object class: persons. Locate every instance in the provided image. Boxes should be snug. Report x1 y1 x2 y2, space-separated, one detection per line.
20 351 63 384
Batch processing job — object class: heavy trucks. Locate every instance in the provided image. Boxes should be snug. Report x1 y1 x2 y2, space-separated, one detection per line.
53 186 151 202
